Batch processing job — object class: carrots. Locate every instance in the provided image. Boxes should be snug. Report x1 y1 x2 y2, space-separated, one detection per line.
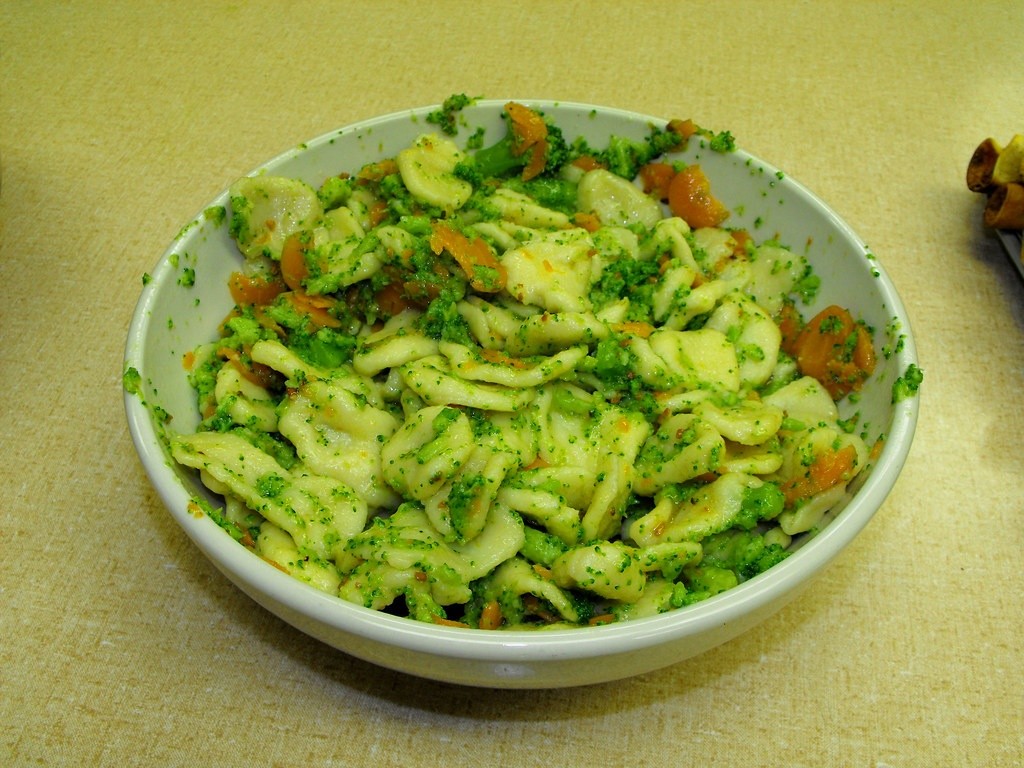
499 102 547 152
668 164 731 229
436 225 509 294
820 328 873 400
279 230 320 291
791 305 856 384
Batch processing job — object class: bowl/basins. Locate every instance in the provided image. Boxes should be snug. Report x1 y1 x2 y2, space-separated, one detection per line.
123 98 920 688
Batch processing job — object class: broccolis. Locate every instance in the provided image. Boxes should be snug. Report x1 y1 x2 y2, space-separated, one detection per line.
453 105 569 183
